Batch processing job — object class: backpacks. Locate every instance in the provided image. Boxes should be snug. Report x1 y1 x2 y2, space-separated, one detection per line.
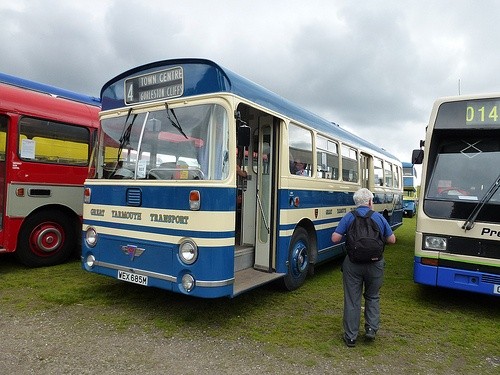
345 209 384 264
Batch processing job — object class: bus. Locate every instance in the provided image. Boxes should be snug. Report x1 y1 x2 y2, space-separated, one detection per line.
412 93 500 297
403 162 420 218
0 73 264 269
81 58 404 300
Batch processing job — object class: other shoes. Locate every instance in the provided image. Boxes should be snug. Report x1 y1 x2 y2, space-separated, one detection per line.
343 333 355 347
365 328 375 339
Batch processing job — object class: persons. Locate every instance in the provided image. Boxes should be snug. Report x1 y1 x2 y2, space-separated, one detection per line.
331 189 397 347
292 156 308 176
198 134 247 181
